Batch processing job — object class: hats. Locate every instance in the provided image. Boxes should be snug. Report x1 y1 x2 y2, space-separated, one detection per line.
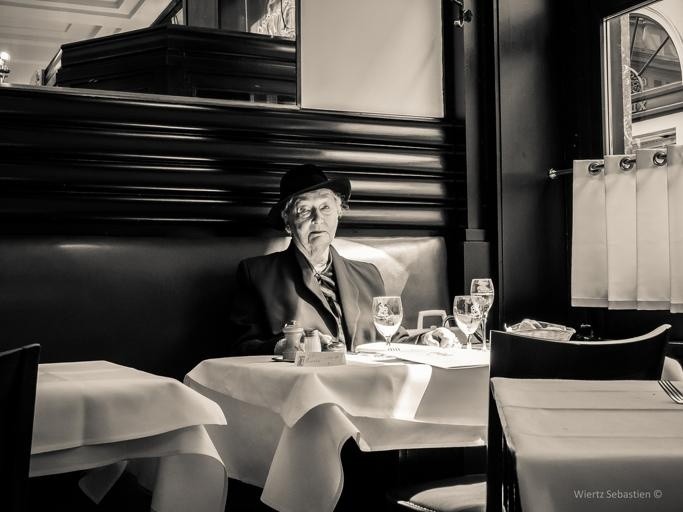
265 163 352 231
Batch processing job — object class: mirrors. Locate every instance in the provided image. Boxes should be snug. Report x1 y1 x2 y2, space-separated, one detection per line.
0 0 301 109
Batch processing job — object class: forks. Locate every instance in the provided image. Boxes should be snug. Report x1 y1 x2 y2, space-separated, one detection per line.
658 380 683 404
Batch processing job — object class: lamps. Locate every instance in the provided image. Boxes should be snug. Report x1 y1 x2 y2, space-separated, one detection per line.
0 50 12 83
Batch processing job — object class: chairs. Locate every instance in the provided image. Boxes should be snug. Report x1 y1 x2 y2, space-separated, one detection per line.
396 325 683 512
0 343 40 511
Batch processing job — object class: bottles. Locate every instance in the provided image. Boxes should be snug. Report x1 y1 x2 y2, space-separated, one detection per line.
304 329 321 352
282 329 302 351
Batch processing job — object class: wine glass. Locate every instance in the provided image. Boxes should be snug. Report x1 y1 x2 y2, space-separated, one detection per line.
470 279 495 350
453 296 483 350
373 296 403 348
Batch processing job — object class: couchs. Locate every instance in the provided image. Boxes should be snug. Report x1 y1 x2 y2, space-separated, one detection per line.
0 235 449 377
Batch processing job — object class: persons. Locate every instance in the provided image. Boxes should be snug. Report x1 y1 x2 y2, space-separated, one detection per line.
230 164 461 354
